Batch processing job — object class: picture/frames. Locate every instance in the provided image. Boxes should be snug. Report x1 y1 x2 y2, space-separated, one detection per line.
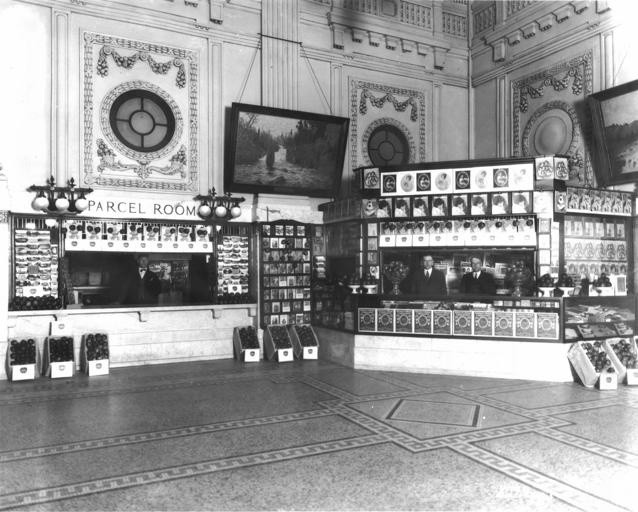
584 79 638 189
220 101 351 200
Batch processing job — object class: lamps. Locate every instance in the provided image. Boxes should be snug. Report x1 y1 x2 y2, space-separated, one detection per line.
192 185 244 226
23 178 97 216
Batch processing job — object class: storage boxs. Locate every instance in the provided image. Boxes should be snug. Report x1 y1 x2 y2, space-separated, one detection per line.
264 323 294 364
79 331 113 376
566 340 625 388
231 323 262 363
2 336 40 382
287 322 320 361
599 335 637 390
40 333 79 380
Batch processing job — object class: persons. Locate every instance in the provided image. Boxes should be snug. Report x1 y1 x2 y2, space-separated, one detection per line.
459 255 497 296
409 253 447 295
112 254 161 305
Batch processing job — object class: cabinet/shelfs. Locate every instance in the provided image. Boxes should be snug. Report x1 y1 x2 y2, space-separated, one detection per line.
259 217 315 329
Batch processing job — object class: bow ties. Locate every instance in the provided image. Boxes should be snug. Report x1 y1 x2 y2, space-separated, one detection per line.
140 269 147 272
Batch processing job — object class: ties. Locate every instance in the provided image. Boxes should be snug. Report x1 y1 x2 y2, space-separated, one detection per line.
426 271 429 279
475 273 477 280
